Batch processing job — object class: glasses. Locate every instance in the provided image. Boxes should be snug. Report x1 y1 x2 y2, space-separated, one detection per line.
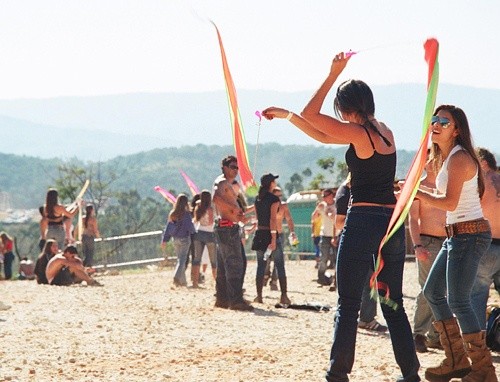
430 115 456 129
228 165 238 170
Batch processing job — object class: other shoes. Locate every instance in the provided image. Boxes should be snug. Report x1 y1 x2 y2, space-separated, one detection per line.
412 333 427 352
253 296 262 303
89 280 105 287
215 297 230 308
318 274 330 284
330 279 336 289
396 377 414 382
281 295 292 304
231 301 254 311
428 335 441 348
173 278 185 286
270 281 278 290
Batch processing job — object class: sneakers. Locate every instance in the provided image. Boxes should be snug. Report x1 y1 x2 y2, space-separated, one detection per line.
358 318 387 335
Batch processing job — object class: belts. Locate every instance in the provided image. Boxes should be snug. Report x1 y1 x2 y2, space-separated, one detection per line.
444 219 491 239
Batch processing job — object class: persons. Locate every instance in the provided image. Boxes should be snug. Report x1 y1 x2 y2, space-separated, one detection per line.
80 204 99 268
192 179 299 291
262 50 424 381
191 191 218 285
251 173 291 308
311 146 499 353
395 104 498 382
18 188 105 287
0 231 14 280
162 194 196 286
214 155 254 311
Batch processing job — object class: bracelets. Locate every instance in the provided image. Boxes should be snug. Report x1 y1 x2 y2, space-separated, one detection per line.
413 244 422 249
286 111 294 122
290 232 296 235
270 231 277 233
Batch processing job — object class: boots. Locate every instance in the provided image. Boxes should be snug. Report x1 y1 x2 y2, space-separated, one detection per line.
448 329 498 382
425 317 471 382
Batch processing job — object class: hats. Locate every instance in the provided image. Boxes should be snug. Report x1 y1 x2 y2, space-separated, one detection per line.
261 174 280 185
